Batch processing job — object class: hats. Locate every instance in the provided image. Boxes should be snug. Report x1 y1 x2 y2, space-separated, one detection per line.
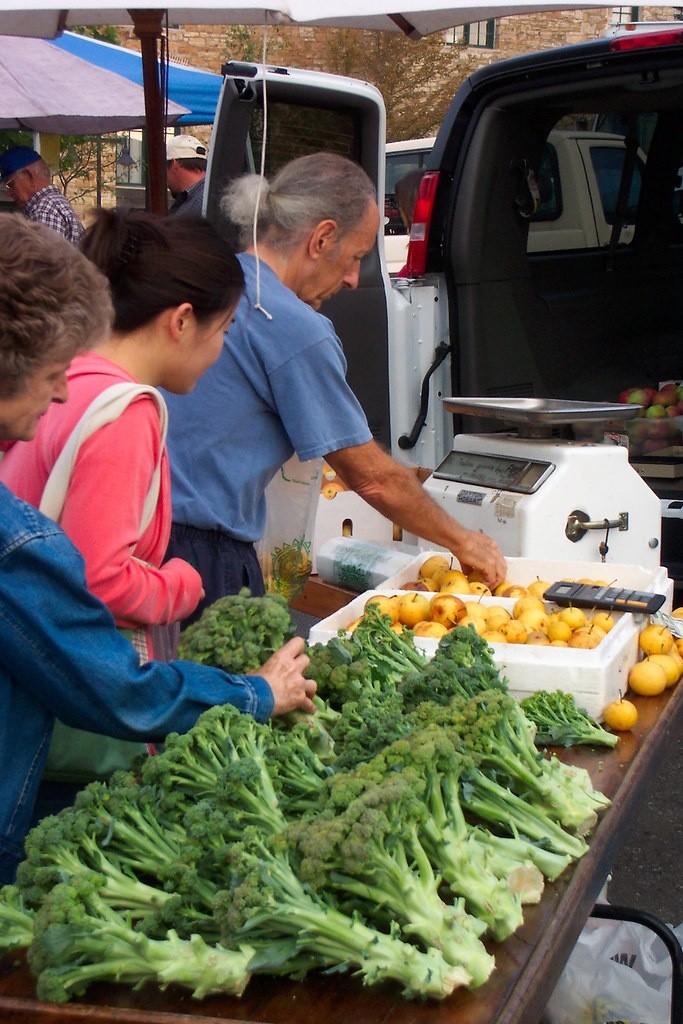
165 134 209 160
0 145 42 183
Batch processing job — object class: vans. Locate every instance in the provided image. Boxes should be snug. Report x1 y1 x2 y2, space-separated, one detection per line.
201 18 682 521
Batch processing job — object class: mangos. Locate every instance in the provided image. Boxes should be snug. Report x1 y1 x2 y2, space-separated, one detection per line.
601 383 683 454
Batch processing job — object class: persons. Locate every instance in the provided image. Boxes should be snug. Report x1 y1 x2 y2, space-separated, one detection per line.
0 145 85 251
0 207 246 836
0 212 318 891
154 150 506 632
166 135 208 217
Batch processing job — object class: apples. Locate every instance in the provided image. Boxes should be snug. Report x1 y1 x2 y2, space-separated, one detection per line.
320 462 348 499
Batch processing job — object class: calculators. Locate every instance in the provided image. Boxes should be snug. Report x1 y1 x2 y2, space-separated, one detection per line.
541 579 667 614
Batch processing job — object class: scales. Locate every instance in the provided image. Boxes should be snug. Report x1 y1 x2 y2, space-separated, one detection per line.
414 394 662 588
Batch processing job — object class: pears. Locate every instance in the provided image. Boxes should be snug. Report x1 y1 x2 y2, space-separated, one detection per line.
342 555 683 730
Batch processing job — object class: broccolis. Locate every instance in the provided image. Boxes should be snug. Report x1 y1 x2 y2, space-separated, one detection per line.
0 586 620 1013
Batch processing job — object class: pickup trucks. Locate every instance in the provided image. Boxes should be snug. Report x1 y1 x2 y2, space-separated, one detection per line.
384 130 647 280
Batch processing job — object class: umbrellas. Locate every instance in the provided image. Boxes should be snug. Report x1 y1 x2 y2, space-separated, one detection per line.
0 34 193 136
42 30 224 126
0 0 683 220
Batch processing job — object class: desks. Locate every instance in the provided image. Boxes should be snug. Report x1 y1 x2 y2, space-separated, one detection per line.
0 572 683 961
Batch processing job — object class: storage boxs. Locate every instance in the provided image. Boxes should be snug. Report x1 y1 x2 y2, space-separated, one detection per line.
375 551 675 622
308 589 650 727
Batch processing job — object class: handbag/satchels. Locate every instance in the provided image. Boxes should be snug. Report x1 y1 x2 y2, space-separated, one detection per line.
255 444 328 607
40 619 156 778
540 876 683 1024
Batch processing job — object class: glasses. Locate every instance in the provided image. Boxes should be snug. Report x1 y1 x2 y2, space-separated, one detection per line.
3 175 19 192
